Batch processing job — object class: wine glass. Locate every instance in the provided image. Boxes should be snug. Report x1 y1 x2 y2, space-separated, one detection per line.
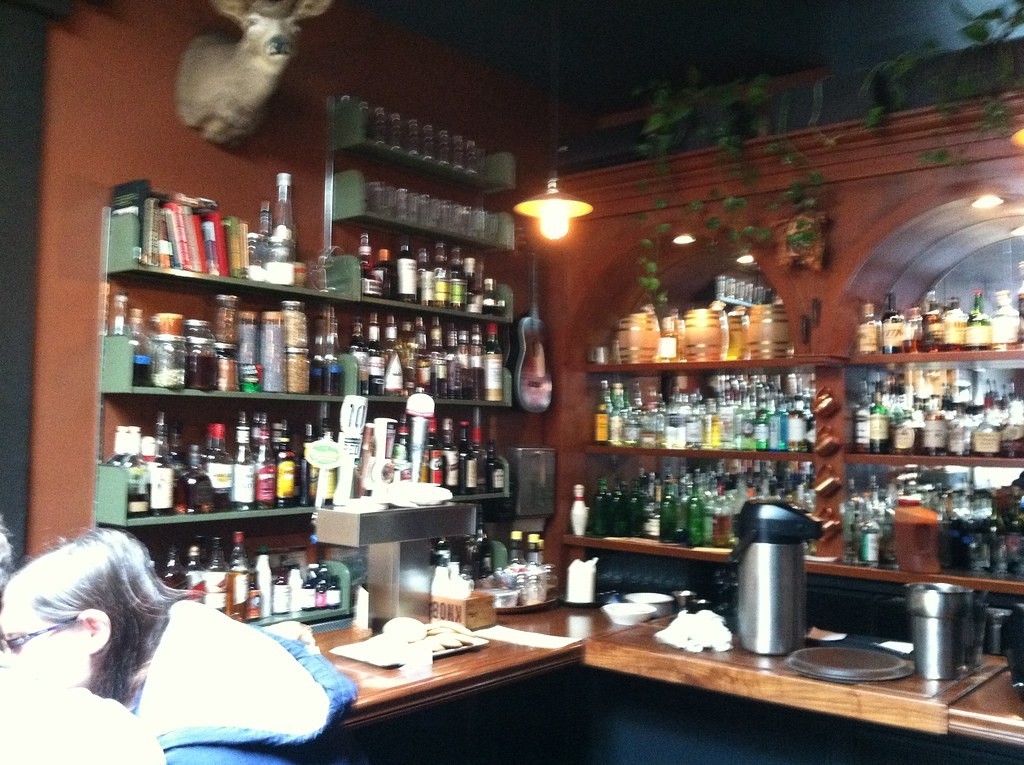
984 606 1012 653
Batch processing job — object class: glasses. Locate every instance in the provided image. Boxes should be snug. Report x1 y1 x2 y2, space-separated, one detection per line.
0 618 81 656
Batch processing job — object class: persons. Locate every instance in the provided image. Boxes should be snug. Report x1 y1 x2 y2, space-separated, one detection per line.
0 527 356 765
0 513 168 765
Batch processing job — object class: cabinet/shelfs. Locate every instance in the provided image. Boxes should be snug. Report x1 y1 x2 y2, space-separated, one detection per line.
83 102 1023 608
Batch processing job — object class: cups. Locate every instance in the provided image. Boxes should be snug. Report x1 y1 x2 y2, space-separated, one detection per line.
961 589 987 667
812 463 841 497
810 386 841 416
514 566 548 605
1008 603 1024 691
814 425 841 457
672 590 711 614
903 581 973 680
816 509 841 543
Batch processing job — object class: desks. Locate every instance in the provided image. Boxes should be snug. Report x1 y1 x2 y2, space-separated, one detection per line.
315 595 1024 765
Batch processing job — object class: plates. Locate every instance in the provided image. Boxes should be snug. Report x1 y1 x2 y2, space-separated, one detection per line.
557 590 623 608
785 647 915 684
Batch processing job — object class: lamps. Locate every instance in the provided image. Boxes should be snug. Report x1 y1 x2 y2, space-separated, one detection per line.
510 131 590 240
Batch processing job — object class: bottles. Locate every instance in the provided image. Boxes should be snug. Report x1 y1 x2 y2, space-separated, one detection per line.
570 262 1024 582
106 94 554 623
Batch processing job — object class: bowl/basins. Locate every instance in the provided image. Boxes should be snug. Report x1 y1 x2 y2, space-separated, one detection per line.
602 593 673 626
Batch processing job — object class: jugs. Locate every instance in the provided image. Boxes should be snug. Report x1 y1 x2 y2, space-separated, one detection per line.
730 500 824 655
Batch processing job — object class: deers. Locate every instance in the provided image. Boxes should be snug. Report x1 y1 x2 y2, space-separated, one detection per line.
171 0 333 148
